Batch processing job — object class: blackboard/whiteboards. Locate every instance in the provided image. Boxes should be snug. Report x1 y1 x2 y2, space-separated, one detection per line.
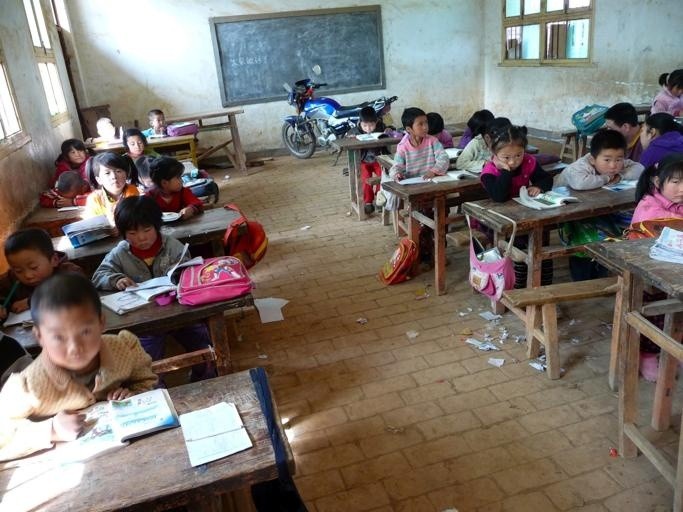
208 5 386 108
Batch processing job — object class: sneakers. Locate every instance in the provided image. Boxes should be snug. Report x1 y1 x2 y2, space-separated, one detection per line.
639 352 660 383
364 204 375 214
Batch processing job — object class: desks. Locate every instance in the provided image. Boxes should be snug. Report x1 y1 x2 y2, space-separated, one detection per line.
581 237 683 512
0 109 248 259
462 185 641 316
327 104 683 294
0 274 255 378
0 369 294 511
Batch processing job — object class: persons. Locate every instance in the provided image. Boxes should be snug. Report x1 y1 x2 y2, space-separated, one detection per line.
0 271 159 464
426 111 454 149
90 196 213 385
630 156 683 381
651 69 682 120
0 332 32 389
388 106 452 269
457 110 554 312
0 228 83 322
554 104 680 281
40 109 202 238
345 105 398 213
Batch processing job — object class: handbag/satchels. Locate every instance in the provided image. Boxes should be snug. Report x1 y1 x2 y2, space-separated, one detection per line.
462 201 518 304
376 154 400 211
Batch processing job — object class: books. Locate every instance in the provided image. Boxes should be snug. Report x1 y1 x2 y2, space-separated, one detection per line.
647 226 680 264
397 175 432 186
123 243 203 291
4 309 32 328
603 179 639 192
99 284 178 315
62 215 113 247
21 320 35 329
512 186 583 211
445 148 464 160
355 130 388 140
67 387 180 464
432 169 478 184
177 401 253 468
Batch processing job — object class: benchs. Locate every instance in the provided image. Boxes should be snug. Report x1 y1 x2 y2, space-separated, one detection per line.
500 278 622 379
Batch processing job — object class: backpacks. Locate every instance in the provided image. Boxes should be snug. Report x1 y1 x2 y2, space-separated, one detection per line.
603 215 682 244
378 237 420 286
222 204 268 270
181 167 219 203
167 121 199 137
571 104 610 140
154 256 257 308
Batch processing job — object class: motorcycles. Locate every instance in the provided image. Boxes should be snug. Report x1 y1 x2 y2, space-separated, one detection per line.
280 64 398 167
